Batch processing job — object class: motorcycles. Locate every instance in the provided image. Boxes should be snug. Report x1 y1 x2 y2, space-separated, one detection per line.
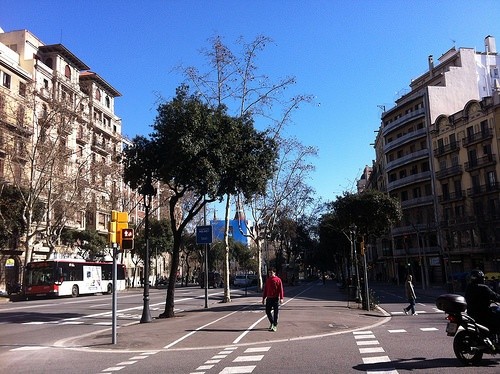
435 293 500 364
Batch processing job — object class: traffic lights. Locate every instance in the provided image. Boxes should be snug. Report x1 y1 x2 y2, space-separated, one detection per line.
108 210 117 243
120 228 134 250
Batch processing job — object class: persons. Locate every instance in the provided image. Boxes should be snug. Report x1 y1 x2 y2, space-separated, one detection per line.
263 267 283 330
402 274 418 316
466 269 500 335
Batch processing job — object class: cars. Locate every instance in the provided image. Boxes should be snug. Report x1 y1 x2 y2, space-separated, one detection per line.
234 275 258 288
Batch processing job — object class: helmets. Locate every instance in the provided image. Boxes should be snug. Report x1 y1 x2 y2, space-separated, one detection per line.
470 270 485 284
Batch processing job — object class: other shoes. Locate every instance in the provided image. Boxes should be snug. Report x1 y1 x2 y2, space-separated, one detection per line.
484 337 496 350
272 327 277 331
269 324 273 330
412 314 417 315
404 309 408 314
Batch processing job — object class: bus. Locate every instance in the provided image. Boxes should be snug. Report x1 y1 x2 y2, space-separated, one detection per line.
21 260 128 298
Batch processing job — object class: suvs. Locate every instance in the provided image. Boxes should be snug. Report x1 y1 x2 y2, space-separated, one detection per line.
198 272 224 288
